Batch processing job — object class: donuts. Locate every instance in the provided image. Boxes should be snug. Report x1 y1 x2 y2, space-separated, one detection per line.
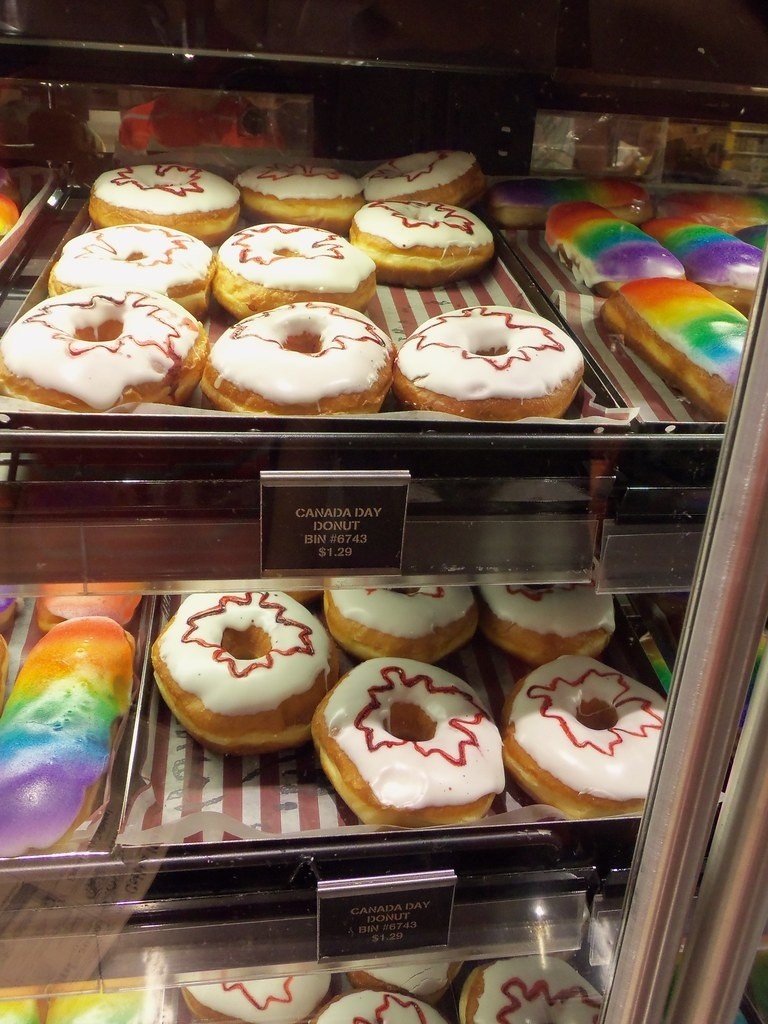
149 581 668 829
172 949 604 1024
0 150 586 423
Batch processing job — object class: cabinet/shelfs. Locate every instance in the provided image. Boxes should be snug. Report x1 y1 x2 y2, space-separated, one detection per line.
0 32 768 1010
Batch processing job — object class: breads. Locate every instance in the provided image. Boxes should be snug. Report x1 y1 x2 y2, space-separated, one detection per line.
0 580 145 860
0 975 155 1023
482 175 767 422
0 167 22 241
